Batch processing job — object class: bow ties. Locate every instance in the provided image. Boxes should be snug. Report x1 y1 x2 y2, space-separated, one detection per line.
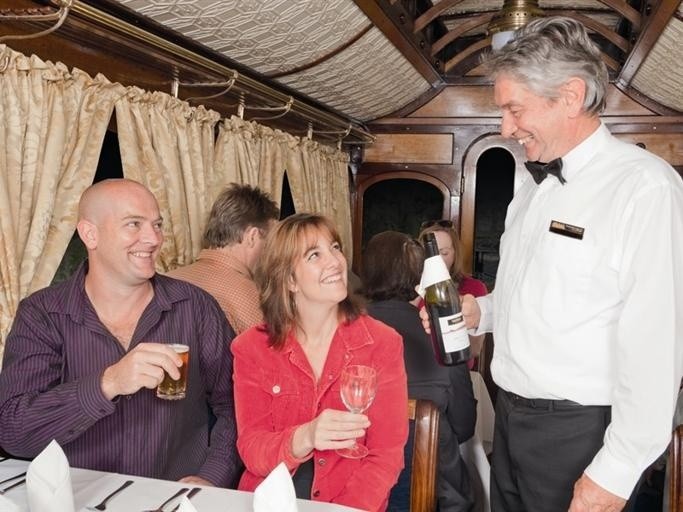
524 157 566 186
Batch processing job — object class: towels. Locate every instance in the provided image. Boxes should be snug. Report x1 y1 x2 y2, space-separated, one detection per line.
253 461 298 512
25 438 74 512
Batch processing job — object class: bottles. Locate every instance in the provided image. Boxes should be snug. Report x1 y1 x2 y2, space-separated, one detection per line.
422 232 474 367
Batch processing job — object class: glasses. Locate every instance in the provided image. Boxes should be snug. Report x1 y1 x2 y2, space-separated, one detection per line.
421 219 454 228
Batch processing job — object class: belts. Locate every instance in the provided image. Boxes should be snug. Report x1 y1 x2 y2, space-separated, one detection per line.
504 391 581 407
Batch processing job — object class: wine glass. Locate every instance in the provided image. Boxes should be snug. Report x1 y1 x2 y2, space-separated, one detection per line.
334 365 378 458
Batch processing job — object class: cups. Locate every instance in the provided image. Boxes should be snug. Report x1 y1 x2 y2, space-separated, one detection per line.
156 344 189 401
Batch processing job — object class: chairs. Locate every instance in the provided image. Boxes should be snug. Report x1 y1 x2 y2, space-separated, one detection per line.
671 425 683 512
385 398 442 512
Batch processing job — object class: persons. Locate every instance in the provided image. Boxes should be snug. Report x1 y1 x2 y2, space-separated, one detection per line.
418 15 683 510
359 220 490 510
0 177 243 488
230 213 409 511
161 184 279 337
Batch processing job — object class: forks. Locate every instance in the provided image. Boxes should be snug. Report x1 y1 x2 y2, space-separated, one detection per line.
85 481 135 512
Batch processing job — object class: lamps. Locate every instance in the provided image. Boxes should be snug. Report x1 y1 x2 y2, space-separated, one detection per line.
486 0 547 54
